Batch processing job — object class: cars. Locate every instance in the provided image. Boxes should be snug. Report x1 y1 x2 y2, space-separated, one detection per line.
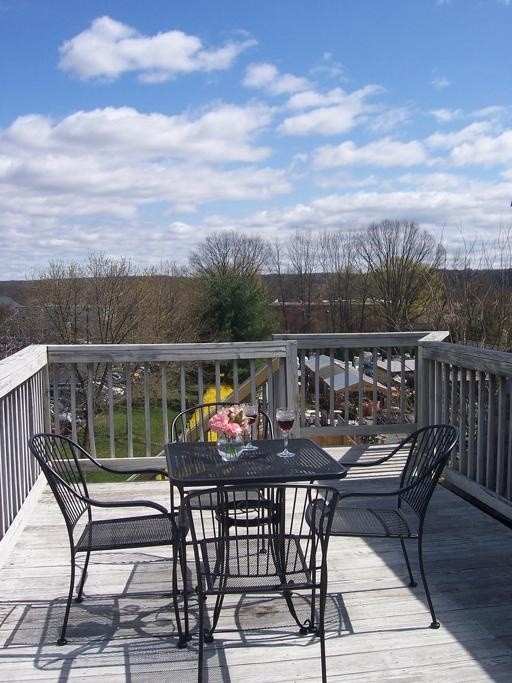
44 363 151 435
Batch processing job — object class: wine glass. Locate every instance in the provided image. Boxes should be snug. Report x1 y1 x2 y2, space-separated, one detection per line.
243 403 258 451
276 407 295 458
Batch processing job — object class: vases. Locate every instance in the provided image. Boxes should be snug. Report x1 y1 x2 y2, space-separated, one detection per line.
215 435 247 463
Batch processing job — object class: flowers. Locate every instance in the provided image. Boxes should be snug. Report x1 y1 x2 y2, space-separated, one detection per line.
207 405 253 443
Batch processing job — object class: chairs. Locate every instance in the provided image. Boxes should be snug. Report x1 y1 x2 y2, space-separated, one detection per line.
181 483 340 683
305 423 459 633
171 403 275 554
28 432 187 649
